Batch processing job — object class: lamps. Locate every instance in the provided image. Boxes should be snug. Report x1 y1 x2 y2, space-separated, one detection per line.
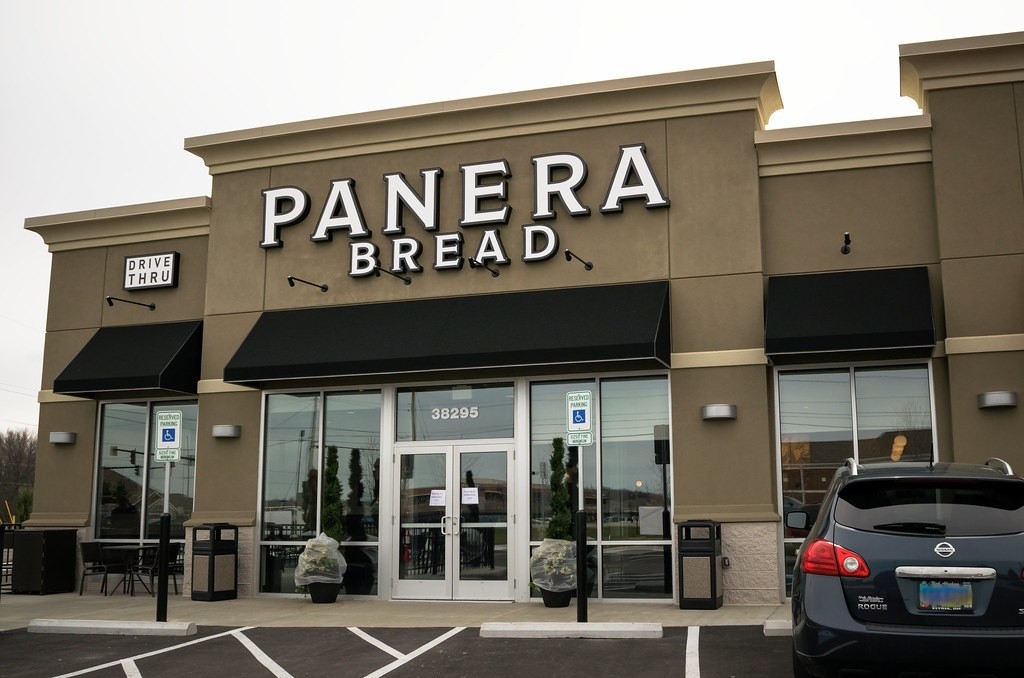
468 256 499 277
564 249 593 271
287 275 329 292
105 295 156 311
841 232 852 254
373 265 411 285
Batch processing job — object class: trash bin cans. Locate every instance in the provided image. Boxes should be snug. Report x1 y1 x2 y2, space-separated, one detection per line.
190 522 239 603
676 520 724 611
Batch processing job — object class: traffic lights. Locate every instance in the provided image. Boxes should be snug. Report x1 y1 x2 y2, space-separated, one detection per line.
129 448 137 465
134 465 139 475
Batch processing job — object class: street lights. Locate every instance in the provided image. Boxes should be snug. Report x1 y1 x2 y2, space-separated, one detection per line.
294 429 308 506
530 470 547 530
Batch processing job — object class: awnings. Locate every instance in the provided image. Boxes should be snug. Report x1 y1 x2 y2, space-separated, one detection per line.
763 267 937 367
222 280 672 389
53 320 199 400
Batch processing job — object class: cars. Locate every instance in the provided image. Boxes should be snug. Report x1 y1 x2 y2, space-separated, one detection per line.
589 539 673 589
478 490 507 515
784 496 809 540
604 516 639 525
406 493 445 511
532 516 553 529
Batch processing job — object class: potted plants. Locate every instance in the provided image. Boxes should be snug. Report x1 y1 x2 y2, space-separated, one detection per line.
529 538 576 608
293 532 348 603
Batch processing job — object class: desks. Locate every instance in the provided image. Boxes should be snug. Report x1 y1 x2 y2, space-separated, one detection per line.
102 544 158 596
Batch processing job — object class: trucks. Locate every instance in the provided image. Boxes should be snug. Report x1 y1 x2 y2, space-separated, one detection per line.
264 506 308 530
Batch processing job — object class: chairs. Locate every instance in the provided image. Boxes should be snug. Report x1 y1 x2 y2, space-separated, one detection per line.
130 543 180 596
80 542 127 596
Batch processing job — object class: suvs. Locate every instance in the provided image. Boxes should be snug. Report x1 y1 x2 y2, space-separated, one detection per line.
788 458 1024 678
